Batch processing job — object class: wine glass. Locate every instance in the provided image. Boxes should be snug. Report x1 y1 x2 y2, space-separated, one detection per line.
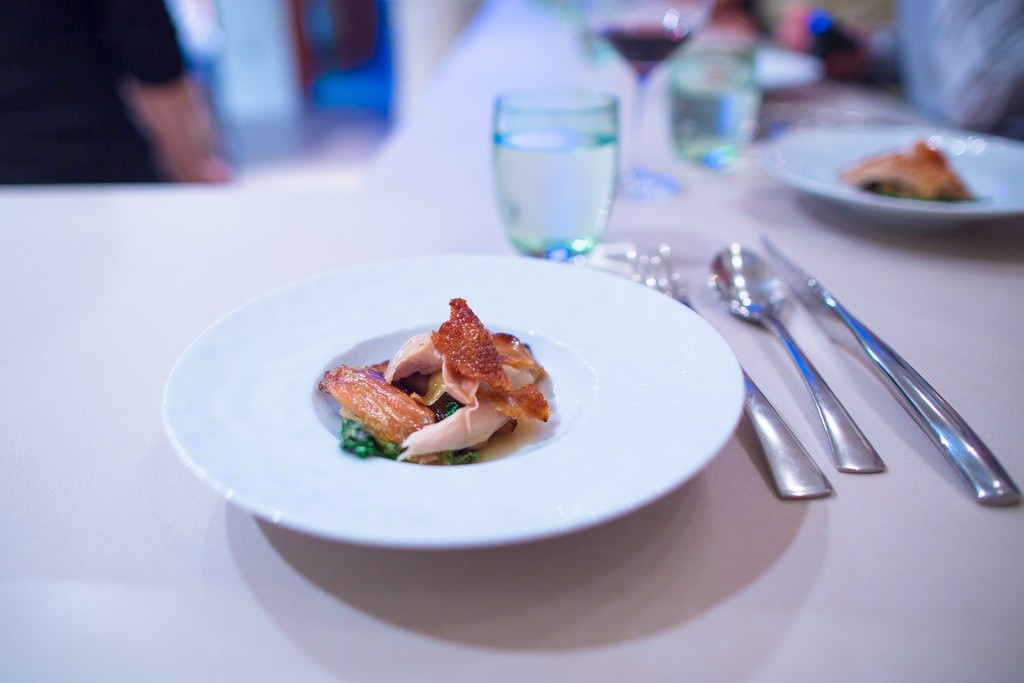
585 0 716 204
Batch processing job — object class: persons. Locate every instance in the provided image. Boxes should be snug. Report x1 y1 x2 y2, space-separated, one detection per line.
777 0 1024 136
0 0 230 186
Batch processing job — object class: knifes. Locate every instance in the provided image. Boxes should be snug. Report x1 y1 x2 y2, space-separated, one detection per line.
759 232 1023 508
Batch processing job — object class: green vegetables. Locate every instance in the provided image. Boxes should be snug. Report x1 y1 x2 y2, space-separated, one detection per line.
338 401 478 464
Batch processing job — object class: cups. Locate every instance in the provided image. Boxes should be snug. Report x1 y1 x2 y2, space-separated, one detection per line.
664 39 762 168
490 91 622 260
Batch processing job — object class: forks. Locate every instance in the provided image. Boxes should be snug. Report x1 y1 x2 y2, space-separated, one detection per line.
625 241 834 501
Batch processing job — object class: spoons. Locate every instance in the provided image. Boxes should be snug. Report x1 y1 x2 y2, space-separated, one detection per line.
704 247 888 474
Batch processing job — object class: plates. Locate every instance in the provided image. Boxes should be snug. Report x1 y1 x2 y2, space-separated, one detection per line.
161 253 746 552
760 46 826 90
764 122 1024 233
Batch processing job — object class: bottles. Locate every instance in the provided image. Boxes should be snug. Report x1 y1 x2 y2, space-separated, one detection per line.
807 9 873 84
